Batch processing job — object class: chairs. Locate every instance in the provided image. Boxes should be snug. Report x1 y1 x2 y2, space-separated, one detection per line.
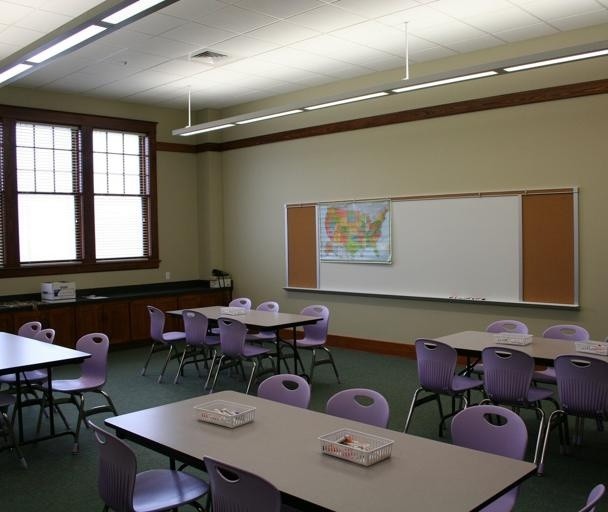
460 320 542 425
578 483 606 512
476 347 561 466
450 406 529 511
85 418 211 512
141 306 202 385
16 321 40 339
202 457 280 511
403 339 484 434
208 317 275 395
208 297 251 374
0 392 29 473
36 331 120 456
257 374 311 411
325 389 390 429
235 301 289 375
537 354 608 475
0 328 55 419
527 324 589 446
172 310 239 389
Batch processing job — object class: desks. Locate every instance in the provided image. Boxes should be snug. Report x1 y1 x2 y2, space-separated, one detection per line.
104 390 537 512
433 330 607 406
0 329 93 462
164 305 324 374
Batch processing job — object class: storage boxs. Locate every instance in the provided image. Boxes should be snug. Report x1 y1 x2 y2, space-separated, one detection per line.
274 306 341 387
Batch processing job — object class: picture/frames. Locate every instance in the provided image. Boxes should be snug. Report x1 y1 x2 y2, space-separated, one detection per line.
315 197 392 266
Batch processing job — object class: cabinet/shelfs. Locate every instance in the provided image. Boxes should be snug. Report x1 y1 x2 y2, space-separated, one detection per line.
179 279 232 332
13 296 76 347
130 287 178 347
75 289 131 350
0 308 13 333
